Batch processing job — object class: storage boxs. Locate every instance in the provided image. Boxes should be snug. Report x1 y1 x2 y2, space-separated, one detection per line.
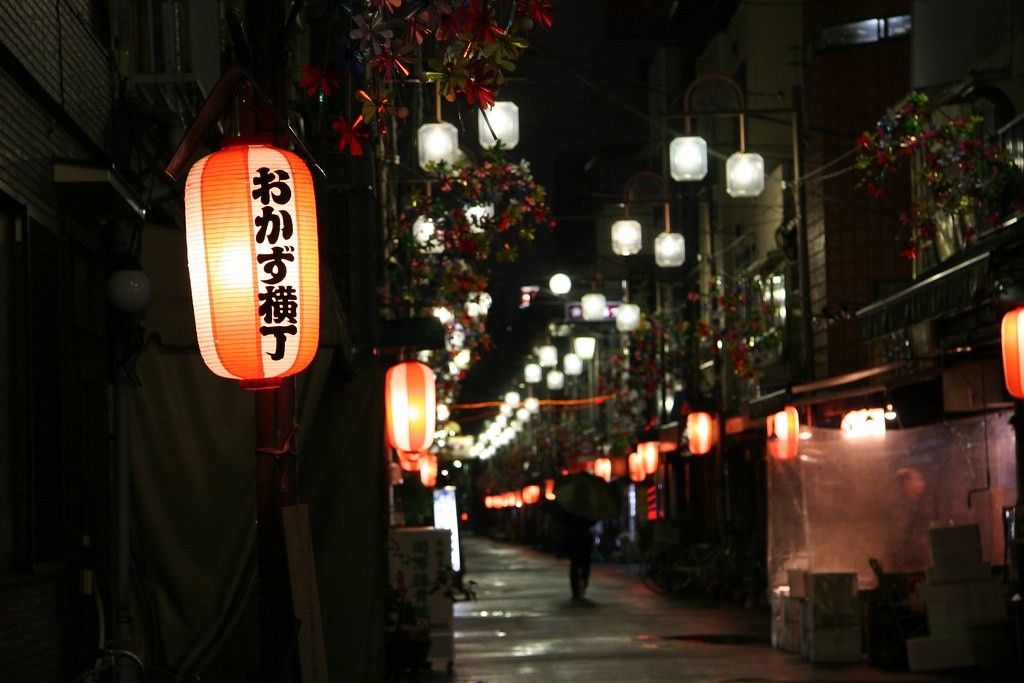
775 571 862 665
904 523 1006 671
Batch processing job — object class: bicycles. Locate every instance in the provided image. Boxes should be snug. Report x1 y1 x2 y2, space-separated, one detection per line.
640 519 754 593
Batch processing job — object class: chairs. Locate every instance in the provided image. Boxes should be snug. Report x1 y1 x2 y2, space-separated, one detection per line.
871 559 928 672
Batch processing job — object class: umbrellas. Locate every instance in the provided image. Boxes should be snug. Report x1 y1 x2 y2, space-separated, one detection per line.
555 471 619 519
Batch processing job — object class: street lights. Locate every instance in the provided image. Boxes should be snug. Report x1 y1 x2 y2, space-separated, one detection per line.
668 73 819 426
579 293 668 424
581 260 669 421
564 328 601 424
377 79 520 316
611 172 729 546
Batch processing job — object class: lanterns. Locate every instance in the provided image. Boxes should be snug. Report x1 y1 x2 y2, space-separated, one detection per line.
627 440 658 482
687 412 712 455
398 449 438 487
385 360 437 453
1000 306 1024 398
184 138 319 390
770 405 799 459
484 480 555 509
594 458 611 482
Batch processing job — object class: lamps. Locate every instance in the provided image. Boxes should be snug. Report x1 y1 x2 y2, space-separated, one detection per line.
615 289 642 331
86 205 183 235
410 181 438 249
652 204 684 267
724 114 765 198
668 117 709 182
416 82 459 173
609 208 643 257
107 221 151 312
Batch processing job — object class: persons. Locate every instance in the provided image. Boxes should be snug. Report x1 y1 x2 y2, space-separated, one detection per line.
546 500 596 607
890 469 941 571
